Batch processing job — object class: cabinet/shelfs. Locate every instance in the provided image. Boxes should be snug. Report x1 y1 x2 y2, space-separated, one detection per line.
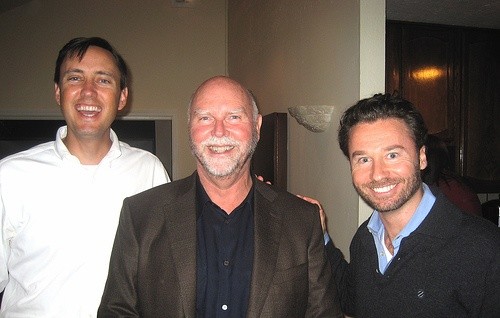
386 19 500 193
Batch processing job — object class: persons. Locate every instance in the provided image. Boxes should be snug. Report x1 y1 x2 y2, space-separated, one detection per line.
0 37 172 318
294 90 500 318
95 76 340 317
